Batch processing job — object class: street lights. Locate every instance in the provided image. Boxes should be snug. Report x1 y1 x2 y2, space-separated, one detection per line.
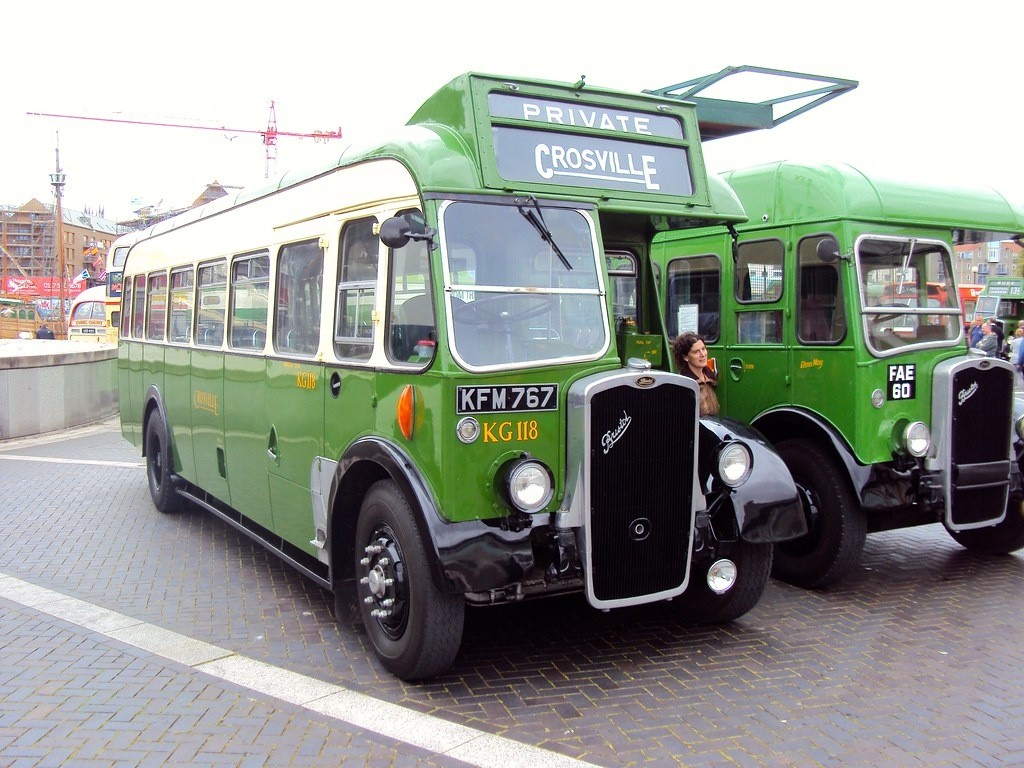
49 148 66 321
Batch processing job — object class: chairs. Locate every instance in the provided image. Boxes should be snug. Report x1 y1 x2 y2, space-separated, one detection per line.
401 296 481 368
775 301 838 345
135 322 319 355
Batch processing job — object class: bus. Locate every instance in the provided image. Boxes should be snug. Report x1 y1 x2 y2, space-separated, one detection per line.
120 73 806 679
67 285 109 344
972 276 1023 355
764 280 885 349
622 61 1018 596
105 229 143 347
883 282 984 343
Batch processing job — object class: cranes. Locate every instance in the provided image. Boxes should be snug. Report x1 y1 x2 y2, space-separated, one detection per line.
28 102 345 183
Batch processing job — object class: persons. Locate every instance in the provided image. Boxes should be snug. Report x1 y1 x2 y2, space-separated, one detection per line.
962 315 1024 381
35 322 55 339
670 331 721 497
